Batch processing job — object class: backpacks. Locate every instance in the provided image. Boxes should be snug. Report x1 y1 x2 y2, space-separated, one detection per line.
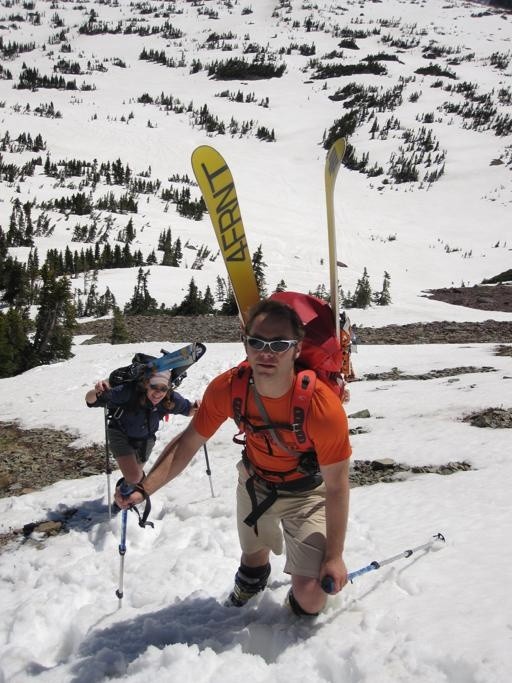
267 292 345 406
109 353 187 389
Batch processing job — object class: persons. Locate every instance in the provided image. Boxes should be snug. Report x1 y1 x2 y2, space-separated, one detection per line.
84 360 204 519
112 299 354 623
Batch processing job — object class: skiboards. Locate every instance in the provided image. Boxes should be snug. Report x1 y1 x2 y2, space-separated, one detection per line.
103 341 206 392
192 138 347 367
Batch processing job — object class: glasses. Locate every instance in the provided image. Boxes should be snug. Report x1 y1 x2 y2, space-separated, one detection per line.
244 335 296 354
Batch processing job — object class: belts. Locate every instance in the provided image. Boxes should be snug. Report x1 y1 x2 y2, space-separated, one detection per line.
241 451 324 494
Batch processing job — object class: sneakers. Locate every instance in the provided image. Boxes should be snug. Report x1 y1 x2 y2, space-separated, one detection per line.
222 565 271 609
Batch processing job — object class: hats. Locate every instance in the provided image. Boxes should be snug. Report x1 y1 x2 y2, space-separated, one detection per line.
149 369 172 388
